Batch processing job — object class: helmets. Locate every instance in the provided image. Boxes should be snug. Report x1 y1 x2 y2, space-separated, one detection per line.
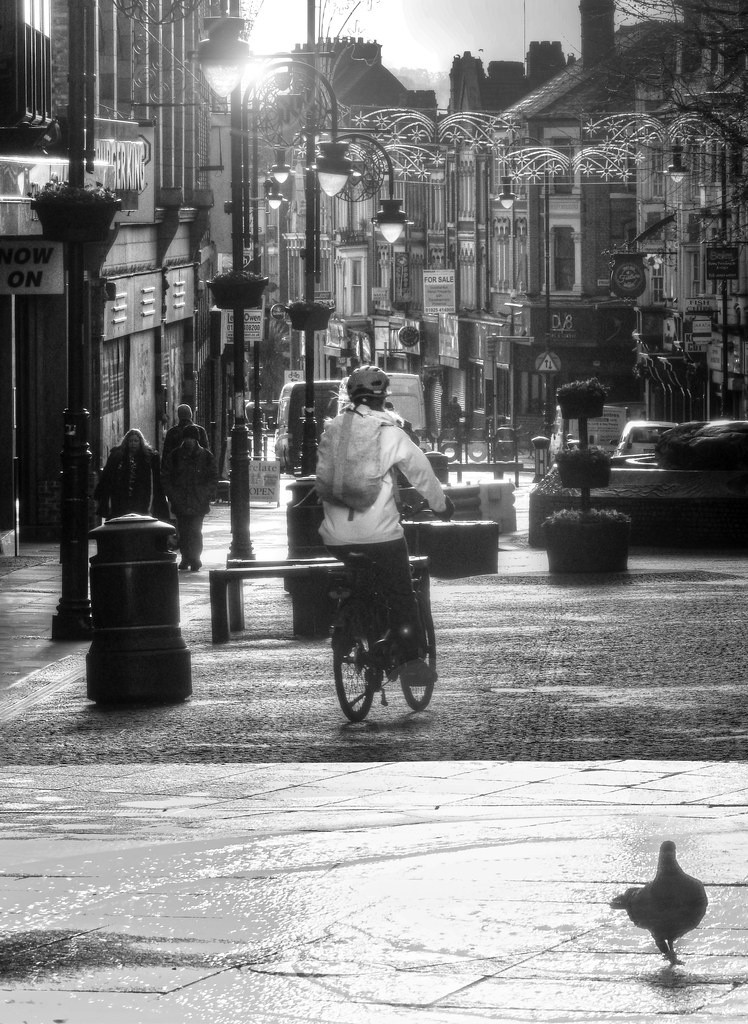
346 366 390 402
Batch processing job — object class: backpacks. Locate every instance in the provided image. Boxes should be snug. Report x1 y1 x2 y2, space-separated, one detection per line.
316 414 394 524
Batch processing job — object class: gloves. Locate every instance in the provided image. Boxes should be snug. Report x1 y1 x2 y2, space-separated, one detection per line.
432 495 454 523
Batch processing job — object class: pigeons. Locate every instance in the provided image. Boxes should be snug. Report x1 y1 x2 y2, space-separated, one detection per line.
609 840 709 965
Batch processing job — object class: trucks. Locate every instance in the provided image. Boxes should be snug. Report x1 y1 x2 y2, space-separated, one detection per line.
273 372 425 474
546 405 626 468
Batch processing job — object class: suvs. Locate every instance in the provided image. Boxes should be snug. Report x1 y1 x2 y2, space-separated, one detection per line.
610 420 679 457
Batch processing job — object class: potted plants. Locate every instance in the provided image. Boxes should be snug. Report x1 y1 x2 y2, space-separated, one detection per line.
556 449 612 488
540 509 630 574
288 300 330 330
557 377 611 420
28 173 122 241
208 270 269 309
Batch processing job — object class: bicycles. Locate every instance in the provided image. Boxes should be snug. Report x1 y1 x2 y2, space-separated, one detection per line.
318 486 438 722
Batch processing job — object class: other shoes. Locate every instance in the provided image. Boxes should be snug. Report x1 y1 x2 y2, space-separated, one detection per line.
179 559 189 569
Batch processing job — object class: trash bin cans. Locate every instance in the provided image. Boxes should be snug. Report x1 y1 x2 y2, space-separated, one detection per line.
496 428 516 461
425 451 448 484
86 512 193 702
284 473 332 591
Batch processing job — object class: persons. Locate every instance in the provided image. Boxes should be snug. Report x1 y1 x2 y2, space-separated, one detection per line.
162 404 210 462
447 396 461 430
384 401 420 446
160 425 218 571
316 364 456 684
93 430 168 522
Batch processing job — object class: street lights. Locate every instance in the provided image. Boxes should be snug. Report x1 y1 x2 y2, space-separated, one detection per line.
668 100 731 420
180 16 408 569
498 138 553 439
